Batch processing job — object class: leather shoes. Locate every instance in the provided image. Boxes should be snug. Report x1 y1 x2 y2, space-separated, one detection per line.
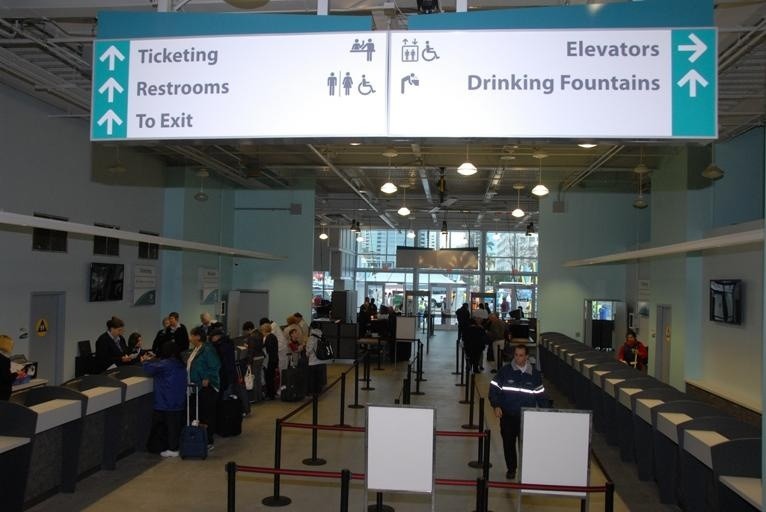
506 468 516 478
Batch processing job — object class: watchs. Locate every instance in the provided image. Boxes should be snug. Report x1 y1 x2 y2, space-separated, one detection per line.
615 331 649 372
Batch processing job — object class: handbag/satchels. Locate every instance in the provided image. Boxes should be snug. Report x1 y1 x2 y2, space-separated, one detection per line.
244 367 255 390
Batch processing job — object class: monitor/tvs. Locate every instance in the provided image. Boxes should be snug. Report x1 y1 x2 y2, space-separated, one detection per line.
508 308 522 320
710 279 742 326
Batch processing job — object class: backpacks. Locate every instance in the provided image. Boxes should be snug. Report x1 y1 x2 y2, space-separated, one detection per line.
310 333 334 360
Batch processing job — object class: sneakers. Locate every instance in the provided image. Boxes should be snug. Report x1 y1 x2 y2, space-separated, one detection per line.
160 450 180 457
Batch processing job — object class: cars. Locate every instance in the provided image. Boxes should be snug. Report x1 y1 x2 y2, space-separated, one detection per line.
312 286 333 296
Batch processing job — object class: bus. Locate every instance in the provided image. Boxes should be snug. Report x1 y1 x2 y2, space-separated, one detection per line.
392 287 447 308
496 281 534 313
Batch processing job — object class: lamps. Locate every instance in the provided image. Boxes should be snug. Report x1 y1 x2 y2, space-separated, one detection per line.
528 221 534 234
317 189 363 244
378 147 401 197
634 148 651 174
511 182 528 219
104 141 128 176
531 151 550 199
698 139 726 185
455 143 480 177
631 178 650 209
524 224 531 237
440 220 448 238
192 169 210 202
396 183 413 215
404 215 416 239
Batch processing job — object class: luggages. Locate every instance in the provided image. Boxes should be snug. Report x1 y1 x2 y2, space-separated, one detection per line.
180 383 207 460
218 397 242 437
280 353 306 402
238 390 250 417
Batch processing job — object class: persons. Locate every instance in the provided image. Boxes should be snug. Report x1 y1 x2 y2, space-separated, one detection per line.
128 332 150 363
440 296 447 325
94 314 134 368
487 344 550 480
518 306 525 318
0 335 26 400
455 302 470 343
359 297 370 316
141 311 335 459
477 302 489 320
500 297 509 319
485 302 492 314
369 298 378 314
